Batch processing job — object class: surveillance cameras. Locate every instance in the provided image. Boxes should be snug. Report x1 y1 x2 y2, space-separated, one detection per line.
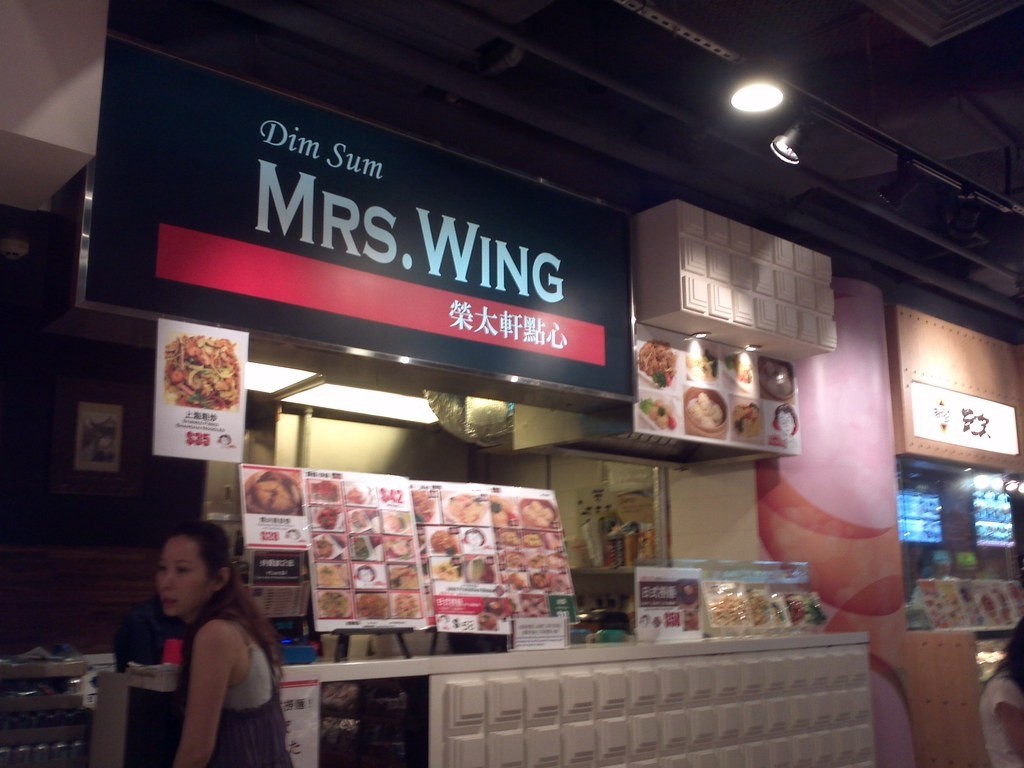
0 231 30 260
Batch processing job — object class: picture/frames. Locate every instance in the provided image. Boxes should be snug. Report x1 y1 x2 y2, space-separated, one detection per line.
74 402 123 473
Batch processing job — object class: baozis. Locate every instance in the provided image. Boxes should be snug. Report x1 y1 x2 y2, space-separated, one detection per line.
688 391 722 429
522 501 554 526
649 399 673 429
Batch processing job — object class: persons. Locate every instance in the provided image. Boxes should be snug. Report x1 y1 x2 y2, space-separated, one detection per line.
978 615 1024 768
155 520 293 768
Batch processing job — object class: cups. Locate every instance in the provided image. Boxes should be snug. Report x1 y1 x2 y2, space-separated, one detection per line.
162 639 183 668
498 550 507 571
608 540 624 566
624 534 638 566
320 634 340 662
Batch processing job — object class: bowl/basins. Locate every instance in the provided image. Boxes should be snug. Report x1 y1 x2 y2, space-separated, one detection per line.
519 499 557 528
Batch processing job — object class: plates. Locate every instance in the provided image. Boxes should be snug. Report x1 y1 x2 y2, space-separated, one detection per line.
345 483 377 507
723 366 754 393
388 565 419 589
431 557 464 586
382 511 414 537
347 509 372 534
315 563 351 588
687 368 720 384
350 536 376 562
317 590 352 618
638 364 680 388
309 481 343 505
390 594 422 619
311 507 344 532
377 486 411 511
386 538 416 563
443 492 491 524
426 526 464 556
639 397 679 431
354 594 389 619
466 556 496 582
313 532 347 562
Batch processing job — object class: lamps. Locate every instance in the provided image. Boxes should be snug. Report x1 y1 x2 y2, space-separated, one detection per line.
770 117 809 165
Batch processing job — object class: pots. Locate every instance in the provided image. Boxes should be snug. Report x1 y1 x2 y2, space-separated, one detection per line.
576 610 629 634
